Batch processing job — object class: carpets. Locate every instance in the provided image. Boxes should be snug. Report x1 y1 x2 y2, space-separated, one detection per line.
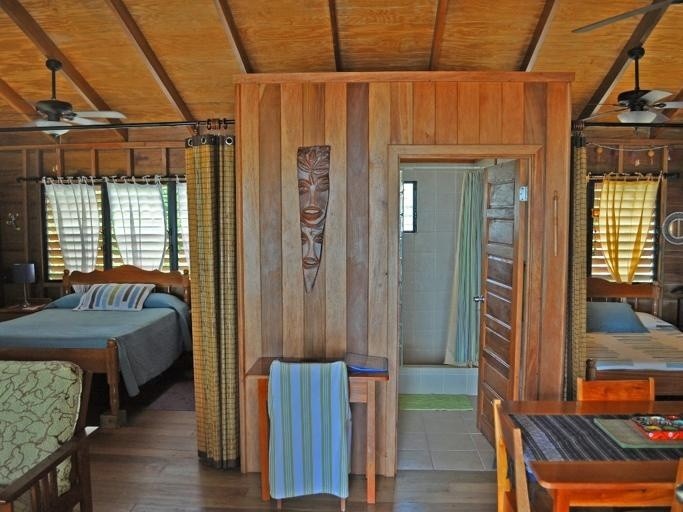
399 393 474 412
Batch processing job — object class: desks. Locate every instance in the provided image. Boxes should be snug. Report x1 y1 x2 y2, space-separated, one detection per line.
502 400 683 512
244 356 390 505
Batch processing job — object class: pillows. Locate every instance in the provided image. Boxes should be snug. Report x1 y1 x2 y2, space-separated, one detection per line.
586 301 664 335
52 283 180 312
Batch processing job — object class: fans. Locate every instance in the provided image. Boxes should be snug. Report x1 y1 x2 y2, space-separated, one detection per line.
579 46 683 132
15 59 126 139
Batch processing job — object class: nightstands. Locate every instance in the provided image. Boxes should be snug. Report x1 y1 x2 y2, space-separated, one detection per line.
1 304 46 322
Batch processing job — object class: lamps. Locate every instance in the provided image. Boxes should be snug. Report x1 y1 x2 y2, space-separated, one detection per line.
13 263 36 308
617 105 657 133
35 113 71 139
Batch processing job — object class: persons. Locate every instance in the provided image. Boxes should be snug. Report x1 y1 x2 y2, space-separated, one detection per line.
299 177 329 270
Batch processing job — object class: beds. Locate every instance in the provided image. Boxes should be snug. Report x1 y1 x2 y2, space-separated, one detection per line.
0 264 191 429
583 276 682 397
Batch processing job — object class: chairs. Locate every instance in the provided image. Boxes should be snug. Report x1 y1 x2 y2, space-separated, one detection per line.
0 360 101 512
269 359 353 512
494 376 658 512
